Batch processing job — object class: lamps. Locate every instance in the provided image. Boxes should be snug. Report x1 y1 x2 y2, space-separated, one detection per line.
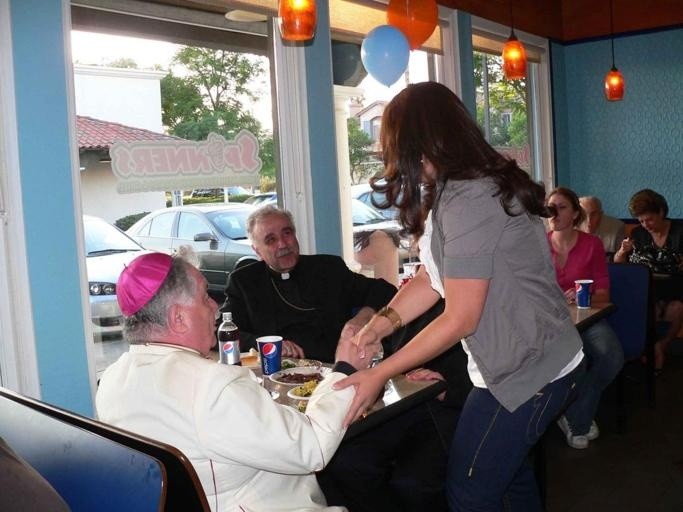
277 0 317 41
501 0 528 80
604 0 624 101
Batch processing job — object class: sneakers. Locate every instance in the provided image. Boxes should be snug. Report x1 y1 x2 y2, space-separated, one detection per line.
558 412 588 450
586 419 599 439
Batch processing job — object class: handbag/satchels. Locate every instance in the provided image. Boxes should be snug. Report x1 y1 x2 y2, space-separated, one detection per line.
626 246 680 277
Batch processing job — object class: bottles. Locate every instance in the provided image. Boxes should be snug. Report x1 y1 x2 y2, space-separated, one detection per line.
217 312 242 367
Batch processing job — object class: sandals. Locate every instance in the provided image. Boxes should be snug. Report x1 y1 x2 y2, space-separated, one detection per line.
652 359 665 377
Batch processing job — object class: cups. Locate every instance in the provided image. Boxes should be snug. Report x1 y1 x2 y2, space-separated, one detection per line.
256 335 284 376
574 279 594 310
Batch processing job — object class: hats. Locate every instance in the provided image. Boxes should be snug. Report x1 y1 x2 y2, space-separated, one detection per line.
115 251 173 314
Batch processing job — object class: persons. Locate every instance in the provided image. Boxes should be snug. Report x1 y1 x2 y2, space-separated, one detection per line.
546 187 682 449
95 83 585 511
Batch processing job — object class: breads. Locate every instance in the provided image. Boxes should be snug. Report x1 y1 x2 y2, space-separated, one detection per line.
239 356 257 366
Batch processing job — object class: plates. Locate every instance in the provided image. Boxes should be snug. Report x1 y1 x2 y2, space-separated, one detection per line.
269 358 333 386
286 385 319 401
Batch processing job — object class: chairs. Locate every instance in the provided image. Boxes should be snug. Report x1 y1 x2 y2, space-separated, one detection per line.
216 220 240 236
0 388 213 512
605 263 651 362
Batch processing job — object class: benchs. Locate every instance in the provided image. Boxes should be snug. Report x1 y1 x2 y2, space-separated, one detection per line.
608 219 642 251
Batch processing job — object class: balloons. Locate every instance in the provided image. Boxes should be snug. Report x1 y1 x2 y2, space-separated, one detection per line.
387 0 439 51
360 25 409 86
